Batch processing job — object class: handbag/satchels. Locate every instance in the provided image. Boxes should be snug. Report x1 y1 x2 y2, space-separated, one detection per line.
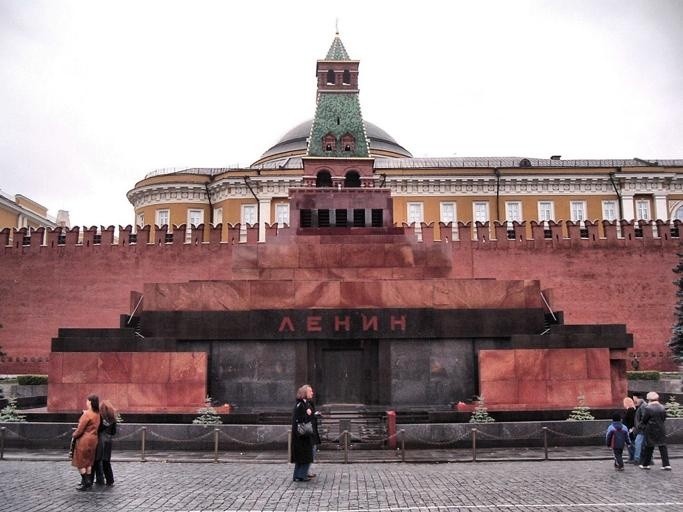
297 421 313 435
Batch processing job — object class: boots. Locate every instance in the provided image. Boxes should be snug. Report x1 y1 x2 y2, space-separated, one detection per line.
76 472 93 491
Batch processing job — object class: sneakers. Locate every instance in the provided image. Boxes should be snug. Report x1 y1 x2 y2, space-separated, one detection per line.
639 465 650 470
660 465 672 471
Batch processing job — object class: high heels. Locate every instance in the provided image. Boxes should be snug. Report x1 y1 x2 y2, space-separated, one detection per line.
293 474 316 481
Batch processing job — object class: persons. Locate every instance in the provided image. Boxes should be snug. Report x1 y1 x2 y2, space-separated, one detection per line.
90 400 117 485
289 383 324 484
602 389 673 473
69 394 98 492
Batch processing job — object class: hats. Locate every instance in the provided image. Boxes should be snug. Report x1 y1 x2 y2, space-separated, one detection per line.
647 392 659 400
